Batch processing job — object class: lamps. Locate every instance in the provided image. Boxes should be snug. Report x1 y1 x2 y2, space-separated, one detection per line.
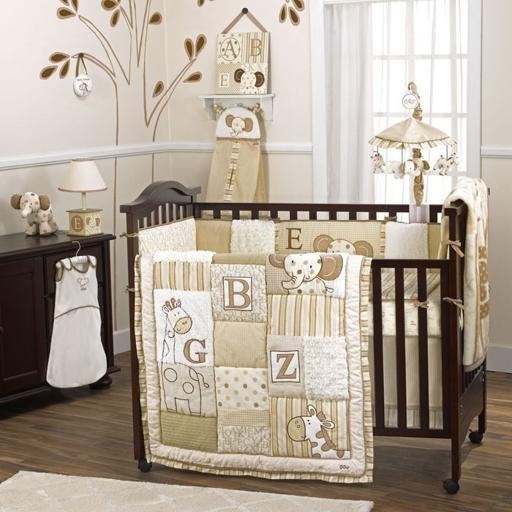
58 158 108 238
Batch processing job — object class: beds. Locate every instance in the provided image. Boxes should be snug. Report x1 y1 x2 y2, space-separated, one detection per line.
120 181 487 494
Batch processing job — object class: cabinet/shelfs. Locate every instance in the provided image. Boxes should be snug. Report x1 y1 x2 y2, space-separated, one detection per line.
0 230 121 404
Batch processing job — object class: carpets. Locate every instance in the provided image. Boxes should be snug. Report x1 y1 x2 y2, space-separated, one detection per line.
0 470 374 512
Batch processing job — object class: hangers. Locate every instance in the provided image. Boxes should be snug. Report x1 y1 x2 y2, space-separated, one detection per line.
55 241 97 268
